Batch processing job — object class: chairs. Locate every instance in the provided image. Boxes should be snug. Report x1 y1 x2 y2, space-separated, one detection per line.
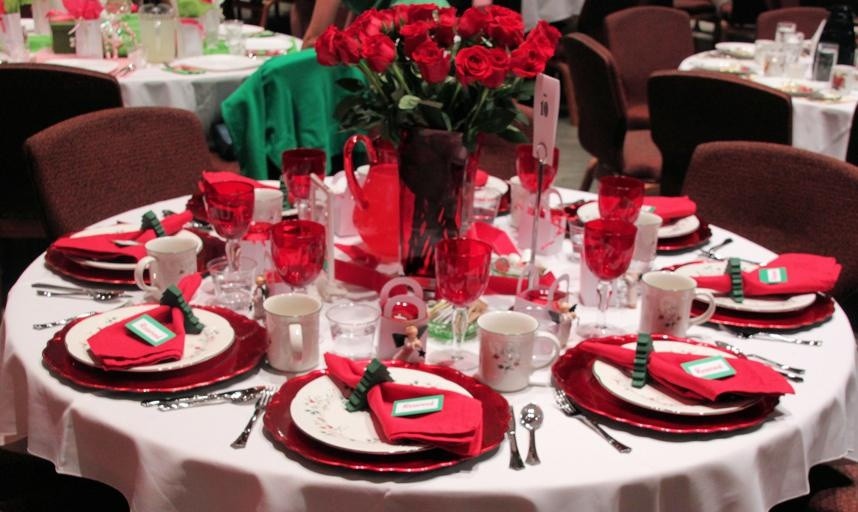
0 5 858 337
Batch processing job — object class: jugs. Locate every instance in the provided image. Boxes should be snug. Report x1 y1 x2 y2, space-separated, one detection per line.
341 134 414 263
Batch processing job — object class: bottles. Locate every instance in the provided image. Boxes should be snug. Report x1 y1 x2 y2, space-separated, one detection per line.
393 127 478 301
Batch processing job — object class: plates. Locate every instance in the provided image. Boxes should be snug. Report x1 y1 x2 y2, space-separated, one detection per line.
713 39 755 55
220 18 293 51
692 56 839 96
553 331 780 434
165 54 262 72
265 355 510 476
42 227 224 290
653 258 835 329
39 300 265 394
35 56 115 73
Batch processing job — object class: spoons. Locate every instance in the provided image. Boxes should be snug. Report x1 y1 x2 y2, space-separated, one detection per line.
519 402 545 466
139 385 270 411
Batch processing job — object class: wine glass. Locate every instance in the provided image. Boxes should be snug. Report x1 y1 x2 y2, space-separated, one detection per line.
270 219 327 295
281 147 328 219
516 142 561 197
597 174 647 222
575 217 637 338
204 180 256 270
427 236 494 371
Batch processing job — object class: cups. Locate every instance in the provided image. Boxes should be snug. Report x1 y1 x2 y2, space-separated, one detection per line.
515 289 573 369
748 19 841 79
640 270 719 335
633 211 664 264
0 1 245 58
264 293 324 372
475 309 561 391
210 256 258 315
325 302 378 358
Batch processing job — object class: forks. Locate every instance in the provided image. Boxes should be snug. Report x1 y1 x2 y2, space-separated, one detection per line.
699 236 733 255
555 389 633 456
31 282 131 299
716 343 806 378
229 390 268 450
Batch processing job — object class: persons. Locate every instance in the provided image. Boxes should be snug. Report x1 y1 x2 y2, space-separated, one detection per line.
302 0 456 67
260 1 314 40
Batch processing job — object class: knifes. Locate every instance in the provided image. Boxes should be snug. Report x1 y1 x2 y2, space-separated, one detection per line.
719 327 822 350
501 402 526 471
